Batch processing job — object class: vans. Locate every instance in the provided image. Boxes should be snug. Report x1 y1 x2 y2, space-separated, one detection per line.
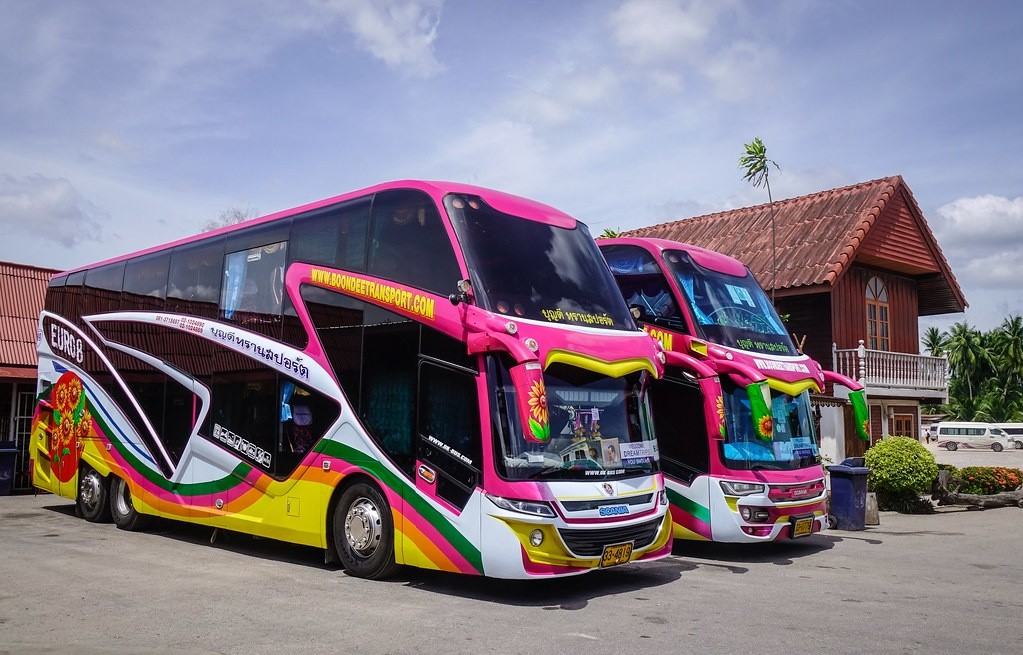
929 421 1023 452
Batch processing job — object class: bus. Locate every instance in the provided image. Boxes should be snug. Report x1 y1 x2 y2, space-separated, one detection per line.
29 179 674 583
598 237 871 545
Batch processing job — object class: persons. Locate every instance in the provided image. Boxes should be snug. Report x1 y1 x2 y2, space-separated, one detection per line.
925 429 930 444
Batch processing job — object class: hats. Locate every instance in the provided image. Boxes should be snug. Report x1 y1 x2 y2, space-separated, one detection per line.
293 406 313 425
244 278 257 295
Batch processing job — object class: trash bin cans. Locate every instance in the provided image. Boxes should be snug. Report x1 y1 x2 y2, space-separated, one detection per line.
825 457 873 531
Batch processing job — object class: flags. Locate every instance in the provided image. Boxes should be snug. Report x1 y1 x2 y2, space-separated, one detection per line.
849 390 871 442
746 381 774 443
